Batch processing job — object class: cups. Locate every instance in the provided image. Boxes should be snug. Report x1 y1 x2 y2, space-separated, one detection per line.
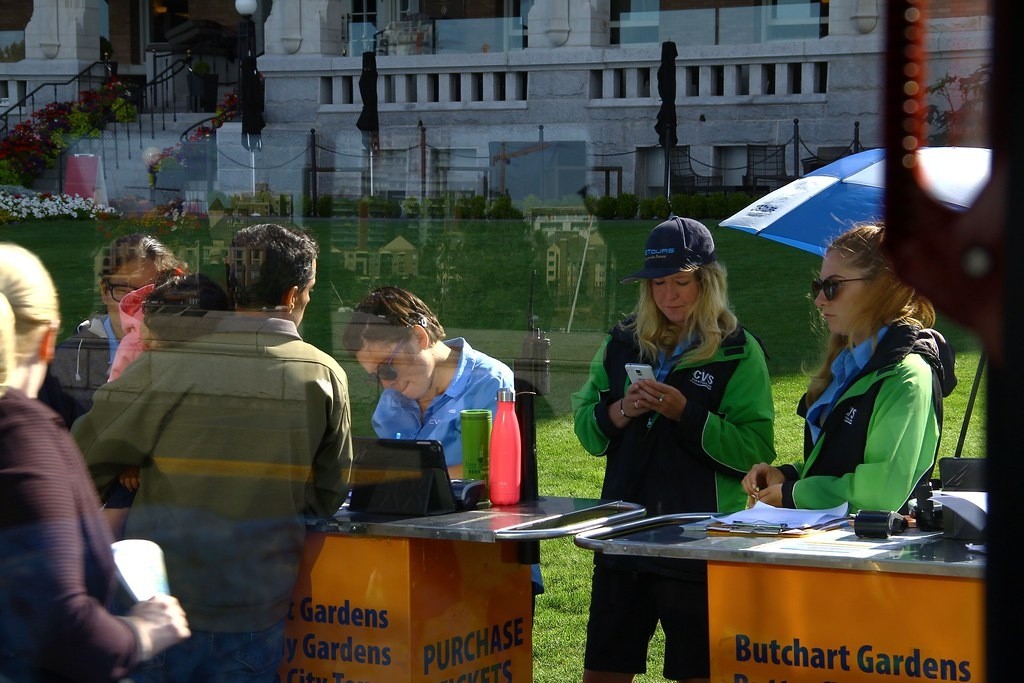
460 410 493 508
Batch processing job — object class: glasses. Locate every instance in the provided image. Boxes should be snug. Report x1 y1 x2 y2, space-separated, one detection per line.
98 274 136 302
811 275 875 301
369 325 415 385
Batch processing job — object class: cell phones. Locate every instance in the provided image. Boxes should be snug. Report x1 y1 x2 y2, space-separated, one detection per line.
623 363 656 384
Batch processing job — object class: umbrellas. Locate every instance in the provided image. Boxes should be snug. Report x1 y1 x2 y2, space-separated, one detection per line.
653 41 679 206
240 20 267 197
356 52 380 196
719 144 991 459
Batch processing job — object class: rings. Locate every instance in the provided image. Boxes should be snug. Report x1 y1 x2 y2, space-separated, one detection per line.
634 402 638 408
660 394 664 402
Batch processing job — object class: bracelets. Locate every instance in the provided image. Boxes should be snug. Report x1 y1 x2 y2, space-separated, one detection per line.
112 616 140 668
620 398 631 419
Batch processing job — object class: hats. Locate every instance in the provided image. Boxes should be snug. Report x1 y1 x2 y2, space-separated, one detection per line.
621 215 717 284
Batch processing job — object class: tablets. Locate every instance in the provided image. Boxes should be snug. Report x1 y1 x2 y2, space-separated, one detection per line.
351 436 459 511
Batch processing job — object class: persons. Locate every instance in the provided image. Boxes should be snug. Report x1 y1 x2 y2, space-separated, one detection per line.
342 285 546 628
96 269 228 535
569 216 777 683
742 225 958 515
0 242 190 683
33 235 183 434
70 224 353 683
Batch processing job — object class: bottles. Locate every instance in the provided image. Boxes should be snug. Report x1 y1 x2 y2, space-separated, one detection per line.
489 387 522 505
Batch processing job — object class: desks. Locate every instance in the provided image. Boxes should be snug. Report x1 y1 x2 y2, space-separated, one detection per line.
278 491 647 683
571 511 987 683
